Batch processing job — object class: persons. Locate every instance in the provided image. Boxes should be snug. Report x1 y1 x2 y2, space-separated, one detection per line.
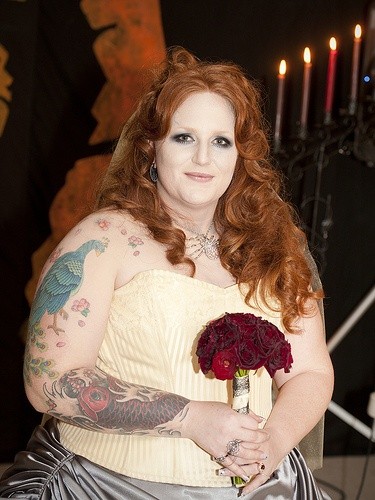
0 45 333 500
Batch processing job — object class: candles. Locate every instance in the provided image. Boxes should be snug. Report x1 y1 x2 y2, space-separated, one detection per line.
274 59 286 153
299 46 312 141
348 24 363 115
326 37 339 126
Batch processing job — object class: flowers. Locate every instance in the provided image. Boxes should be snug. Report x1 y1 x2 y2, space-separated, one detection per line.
197 312 295 489
